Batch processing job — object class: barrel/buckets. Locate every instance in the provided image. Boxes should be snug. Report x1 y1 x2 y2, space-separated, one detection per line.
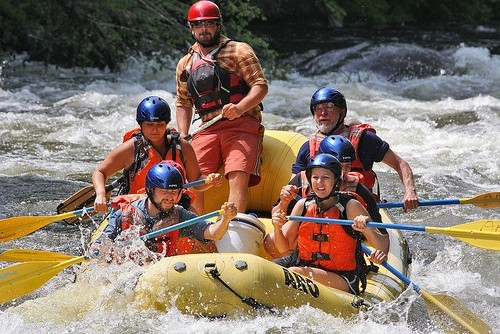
214 213 267 256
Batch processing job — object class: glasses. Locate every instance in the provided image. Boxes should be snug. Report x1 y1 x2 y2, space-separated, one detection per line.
191 21 216 27
311 102 337 113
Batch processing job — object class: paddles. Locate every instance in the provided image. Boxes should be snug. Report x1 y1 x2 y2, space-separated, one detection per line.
377 192 499 209
57 114 223 227
0 249 90 263
0 173 215 242
286 216 500 249
292 193 493 334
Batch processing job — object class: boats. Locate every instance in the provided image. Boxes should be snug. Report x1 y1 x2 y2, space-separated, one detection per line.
79 129 413 321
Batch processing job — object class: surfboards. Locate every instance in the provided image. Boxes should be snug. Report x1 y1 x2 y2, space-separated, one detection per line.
1 202 228 303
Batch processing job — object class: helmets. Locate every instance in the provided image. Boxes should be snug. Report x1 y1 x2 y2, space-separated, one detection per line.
318 134 356 163
309 87 347 114
305 154 344 189
135 96 172 122
187 0 221 21
145 163 183 189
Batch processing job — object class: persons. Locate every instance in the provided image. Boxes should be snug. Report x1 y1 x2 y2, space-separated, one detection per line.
175 0 268 215
89 164 237 260
272 135 390 269
92 97 222 213
288 87 419 213
273 154 386 295
